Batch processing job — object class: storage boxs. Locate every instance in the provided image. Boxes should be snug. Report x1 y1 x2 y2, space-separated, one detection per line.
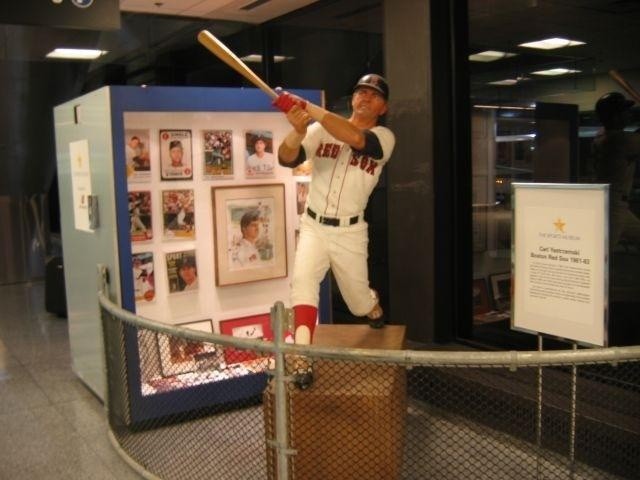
266 324 406 480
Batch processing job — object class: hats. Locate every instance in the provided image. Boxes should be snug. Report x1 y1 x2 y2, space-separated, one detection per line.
176 257 195 270
169 141 182 150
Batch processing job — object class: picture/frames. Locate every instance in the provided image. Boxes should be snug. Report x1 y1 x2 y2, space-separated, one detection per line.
210 181 288 287
219 315 274 365
157 318 218 379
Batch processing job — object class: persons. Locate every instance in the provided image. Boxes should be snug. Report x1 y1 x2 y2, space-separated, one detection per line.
272 74 396 390
126 127 272 303
590 92 640 252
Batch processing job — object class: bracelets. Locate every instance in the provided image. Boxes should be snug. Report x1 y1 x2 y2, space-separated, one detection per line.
305 100 328 123
284 128 306 150
